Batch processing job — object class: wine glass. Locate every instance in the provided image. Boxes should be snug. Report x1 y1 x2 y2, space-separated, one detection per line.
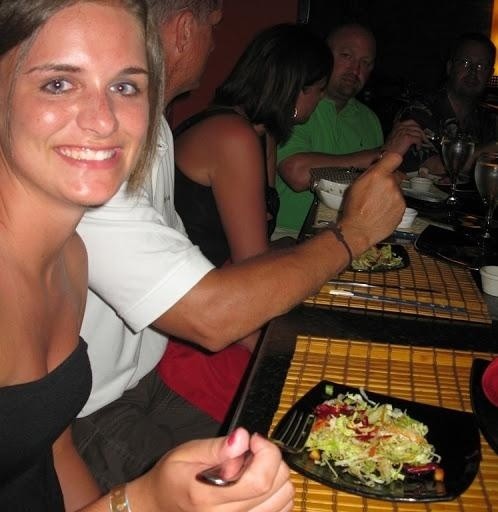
437 136 476 212
469 151 498 244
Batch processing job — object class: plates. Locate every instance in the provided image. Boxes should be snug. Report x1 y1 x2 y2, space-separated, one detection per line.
406 169 471 186
345 242 410 274
413 223 498 272
269 378 483 504
469 358 498 456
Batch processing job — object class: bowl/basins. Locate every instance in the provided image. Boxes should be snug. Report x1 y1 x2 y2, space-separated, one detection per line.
396 207 419 229
479 266 498 298
315 178 353 213
409 176 434 193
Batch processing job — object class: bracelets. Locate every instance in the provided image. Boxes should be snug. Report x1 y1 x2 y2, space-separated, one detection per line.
328 217 355 270
108 482 132 512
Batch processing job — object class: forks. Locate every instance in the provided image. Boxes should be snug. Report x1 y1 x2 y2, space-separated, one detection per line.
194 409 316 489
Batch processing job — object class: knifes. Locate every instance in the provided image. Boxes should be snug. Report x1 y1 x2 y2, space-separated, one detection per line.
327 278 443 296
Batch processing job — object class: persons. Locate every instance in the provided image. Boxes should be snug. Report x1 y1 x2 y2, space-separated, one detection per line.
267 22 422 237
0 0 302 512
157 22 337 424
70 0 408 498
391 31 497 175
